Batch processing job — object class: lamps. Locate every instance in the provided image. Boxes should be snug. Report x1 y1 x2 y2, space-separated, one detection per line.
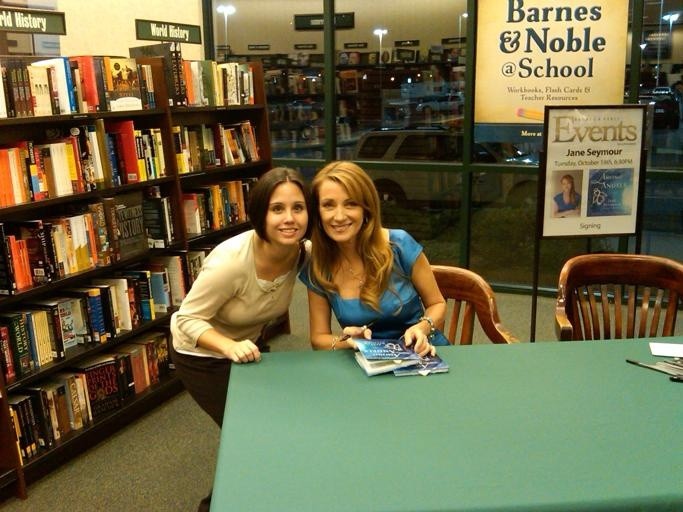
553 252 682 340
414 264 524 344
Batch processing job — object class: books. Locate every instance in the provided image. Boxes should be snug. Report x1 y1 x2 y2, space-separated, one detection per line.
392 354 449 375
171 119 260 174
182 176 259 234
1 186 175 296
9 324 176 459
189 243 217 282
0 250 187 379
351 337 422 376
0 57 156 117
261 45 467 156
129 42 254 106
0 118 168 207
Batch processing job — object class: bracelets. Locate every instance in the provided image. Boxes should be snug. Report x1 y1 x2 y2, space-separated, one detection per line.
420 319 436 339
330 335 340 350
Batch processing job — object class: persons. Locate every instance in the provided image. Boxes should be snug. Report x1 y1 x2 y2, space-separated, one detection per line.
170 167 311 511
304 160 448 358
551 173 580 220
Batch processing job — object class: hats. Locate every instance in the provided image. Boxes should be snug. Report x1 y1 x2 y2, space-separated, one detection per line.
622 84 682 131
643 149 683 214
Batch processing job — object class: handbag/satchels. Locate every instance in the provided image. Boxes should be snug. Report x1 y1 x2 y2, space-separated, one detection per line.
330 335 340 350
420 319 436 339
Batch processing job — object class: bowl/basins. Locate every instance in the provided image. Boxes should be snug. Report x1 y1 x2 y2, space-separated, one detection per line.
344 257 371 288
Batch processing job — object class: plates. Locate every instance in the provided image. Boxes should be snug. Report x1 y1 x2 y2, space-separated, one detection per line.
0 55 185 502
152 56 292 406
270 62 463 160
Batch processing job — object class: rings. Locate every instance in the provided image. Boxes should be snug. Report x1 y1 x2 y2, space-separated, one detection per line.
669 375 683 383
517 107 545 121
340 322 374 342
625 358 683 376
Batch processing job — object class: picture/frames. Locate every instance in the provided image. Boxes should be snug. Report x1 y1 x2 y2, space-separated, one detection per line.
206 338 682 512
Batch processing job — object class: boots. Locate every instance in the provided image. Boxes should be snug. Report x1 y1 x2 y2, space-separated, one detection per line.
349 127 540 213
385 91 464 117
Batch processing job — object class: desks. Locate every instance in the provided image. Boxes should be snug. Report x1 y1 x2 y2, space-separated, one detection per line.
662 9 681 51
216 3 235 61
371 28 387 93
455 11 468 50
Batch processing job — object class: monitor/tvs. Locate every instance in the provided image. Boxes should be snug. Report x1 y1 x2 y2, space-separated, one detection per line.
261 45 467 156
351 337 422 376
392 354 449 375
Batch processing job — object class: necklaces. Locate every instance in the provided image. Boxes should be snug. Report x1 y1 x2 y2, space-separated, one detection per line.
344 257 371 288
